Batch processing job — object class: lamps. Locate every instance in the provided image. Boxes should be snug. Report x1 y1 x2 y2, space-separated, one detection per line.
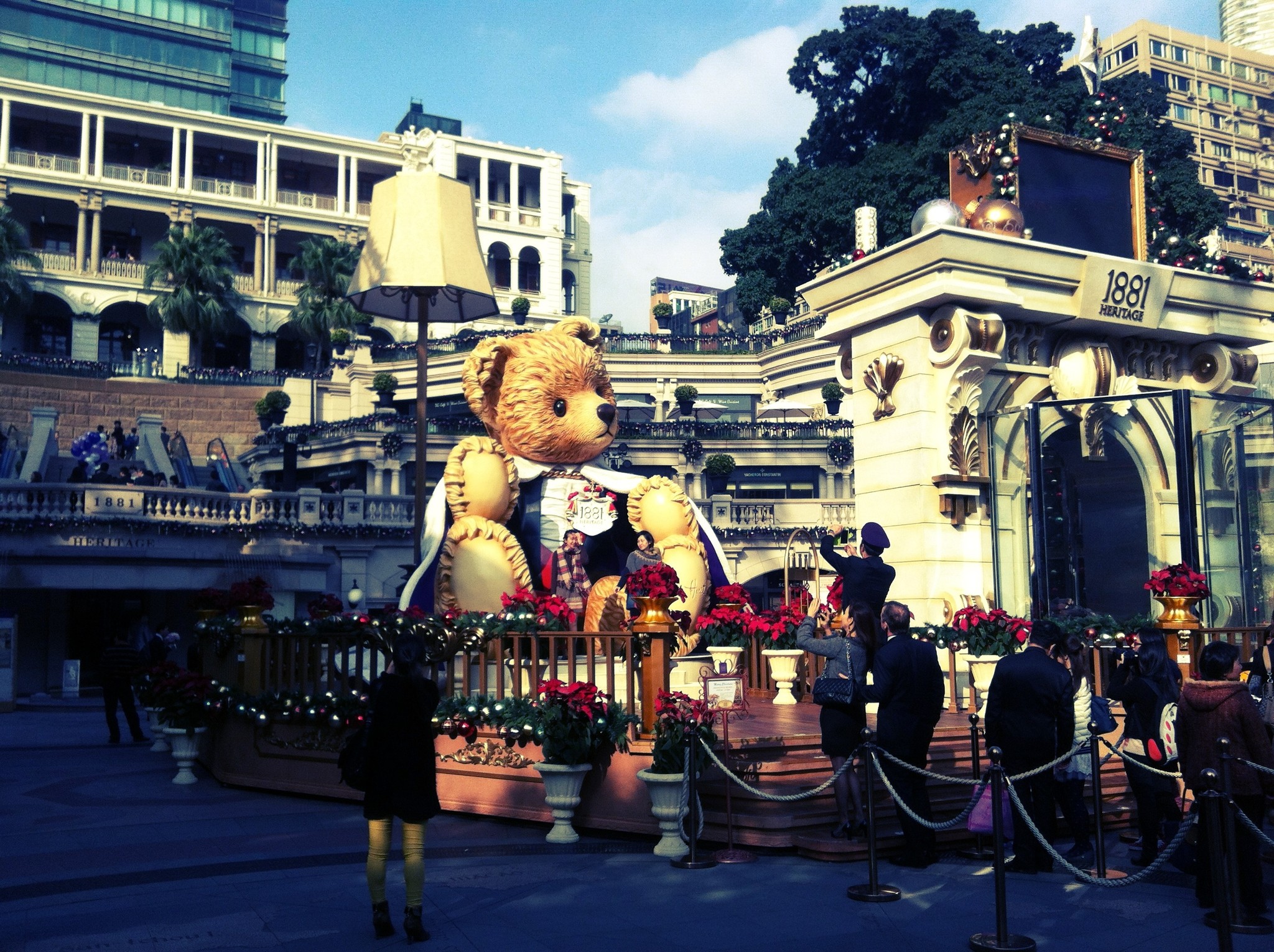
601 443 632 471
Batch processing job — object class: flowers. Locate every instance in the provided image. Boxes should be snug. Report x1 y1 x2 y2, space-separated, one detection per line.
1144 559 1211 600
308 585 576 637
952 605 1032 658
230 574 275 613
135 659 231 736
624 561 843 650
535 678 718 775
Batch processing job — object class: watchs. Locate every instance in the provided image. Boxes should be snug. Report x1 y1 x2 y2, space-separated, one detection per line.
828 529 834 534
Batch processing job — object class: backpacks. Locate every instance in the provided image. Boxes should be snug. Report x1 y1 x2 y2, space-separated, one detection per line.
1138 676 1179 767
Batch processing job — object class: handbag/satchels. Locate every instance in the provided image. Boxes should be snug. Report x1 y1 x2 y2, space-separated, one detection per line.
812 678 854 706
1247 674 1274 728
337 721 374 791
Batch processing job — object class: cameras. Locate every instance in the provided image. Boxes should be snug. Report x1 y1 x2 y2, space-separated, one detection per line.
1112 648 1135 661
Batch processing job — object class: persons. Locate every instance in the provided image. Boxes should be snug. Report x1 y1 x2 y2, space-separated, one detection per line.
106 245 119 259
98 629 150 744
984 621 1092 874
55 431 59 441
325 478 356 520
356 638 440 945
820 523 895 684
549 530 591 646
25 472 44 509
160 427 170 451
1106 609 1274 908
97 420 139 460
614 530 662 618
67 461 245 515
128 253 134 260
797 600 875 839
149 622 176 669
840 601 945 868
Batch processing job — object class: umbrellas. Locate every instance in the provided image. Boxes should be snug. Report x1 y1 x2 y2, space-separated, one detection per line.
757 400 815 422
616 398 656 422
666 401 729 422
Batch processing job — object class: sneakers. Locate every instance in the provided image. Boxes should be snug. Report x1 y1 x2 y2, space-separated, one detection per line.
1129 835 1143 851
1156 839 1168 853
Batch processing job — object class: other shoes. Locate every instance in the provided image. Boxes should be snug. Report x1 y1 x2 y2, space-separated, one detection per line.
1059 843 1095 863
1002 855 1054 874
134 736 150 743
108 739 120 744
891 849 936 868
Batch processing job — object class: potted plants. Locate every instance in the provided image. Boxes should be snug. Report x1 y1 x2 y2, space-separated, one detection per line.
653 302 735 493
511 297 531 326
770 297 847 416
373 372 398 407
255 391 291 432
330 328 350 355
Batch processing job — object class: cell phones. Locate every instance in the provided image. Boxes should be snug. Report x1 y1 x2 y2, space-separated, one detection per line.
840 528 849 545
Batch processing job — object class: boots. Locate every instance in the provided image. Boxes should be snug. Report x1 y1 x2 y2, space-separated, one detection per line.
1131 818 1159 867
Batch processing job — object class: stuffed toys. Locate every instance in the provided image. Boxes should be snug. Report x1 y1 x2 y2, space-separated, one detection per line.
397 317 710 656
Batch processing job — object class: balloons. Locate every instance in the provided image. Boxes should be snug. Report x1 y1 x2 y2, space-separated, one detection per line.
70 430 108 480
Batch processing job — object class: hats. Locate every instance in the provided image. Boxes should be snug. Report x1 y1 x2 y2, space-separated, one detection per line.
862 522 890 551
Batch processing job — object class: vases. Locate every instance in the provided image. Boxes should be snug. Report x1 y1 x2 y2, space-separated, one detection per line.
631 597 679 626
144 707 170 751
534 762 692 858
1152 596 1202 623
161 726 203 786
960 654 1007 718
832 609 843 624
238 605 269 629
706 645 804 705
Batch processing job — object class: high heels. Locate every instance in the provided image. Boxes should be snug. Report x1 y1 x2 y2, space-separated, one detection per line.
832 823 852 840
372 900 395 939
404 905 431 944
853 821 868 838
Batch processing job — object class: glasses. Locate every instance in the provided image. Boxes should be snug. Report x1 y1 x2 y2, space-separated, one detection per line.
1134 639 1142 647
